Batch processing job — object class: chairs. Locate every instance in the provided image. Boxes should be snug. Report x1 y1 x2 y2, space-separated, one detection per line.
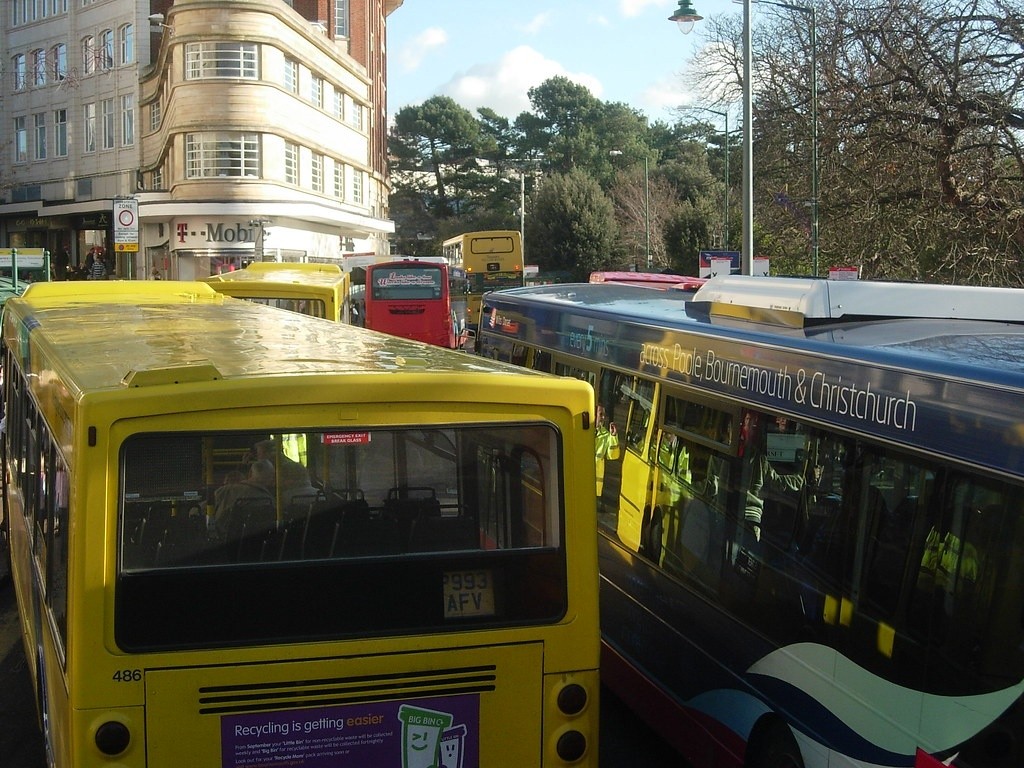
684 478 922 612
126 484 485 575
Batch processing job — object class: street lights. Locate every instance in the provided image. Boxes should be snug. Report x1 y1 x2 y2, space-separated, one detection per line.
670 0 820 280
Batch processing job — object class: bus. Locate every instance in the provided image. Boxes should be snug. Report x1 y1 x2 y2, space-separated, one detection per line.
478 260 1024 767
193 259 352 329
363 258 470 347
439 228 525 336
0 275 609 767
586 268 710 294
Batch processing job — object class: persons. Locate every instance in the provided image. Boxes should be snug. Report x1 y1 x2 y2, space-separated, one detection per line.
625 398 1023 684
593 403 622 498
0 357 75 540
0 244 109 284
205 439 328 549
